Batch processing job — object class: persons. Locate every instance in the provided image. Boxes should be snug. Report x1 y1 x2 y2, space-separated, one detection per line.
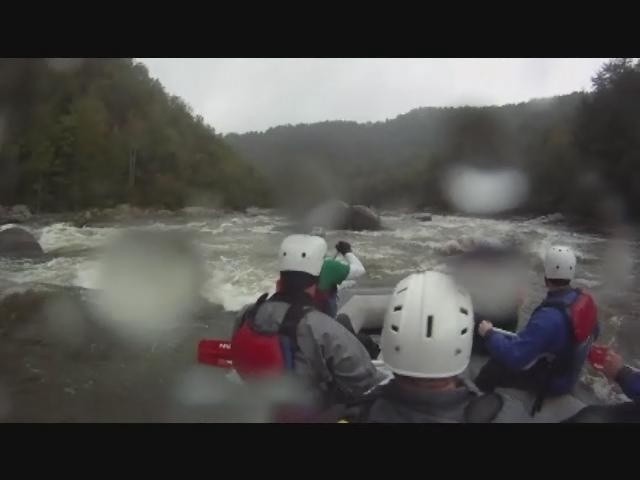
560 343 639 423
274 240 381 359
457 245 518 356
232 234 388 424
348 271 533 423
468 244 600 394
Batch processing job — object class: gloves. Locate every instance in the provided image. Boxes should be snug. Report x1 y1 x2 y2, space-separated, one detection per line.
335 241 352 255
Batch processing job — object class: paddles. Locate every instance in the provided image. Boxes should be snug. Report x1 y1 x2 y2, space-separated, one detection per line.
198 339 384 369
478 320 611 371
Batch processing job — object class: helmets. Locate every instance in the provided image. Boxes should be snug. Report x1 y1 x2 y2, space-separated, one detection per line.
544 245 575 280
279 234 326 276
379 271 474 378
